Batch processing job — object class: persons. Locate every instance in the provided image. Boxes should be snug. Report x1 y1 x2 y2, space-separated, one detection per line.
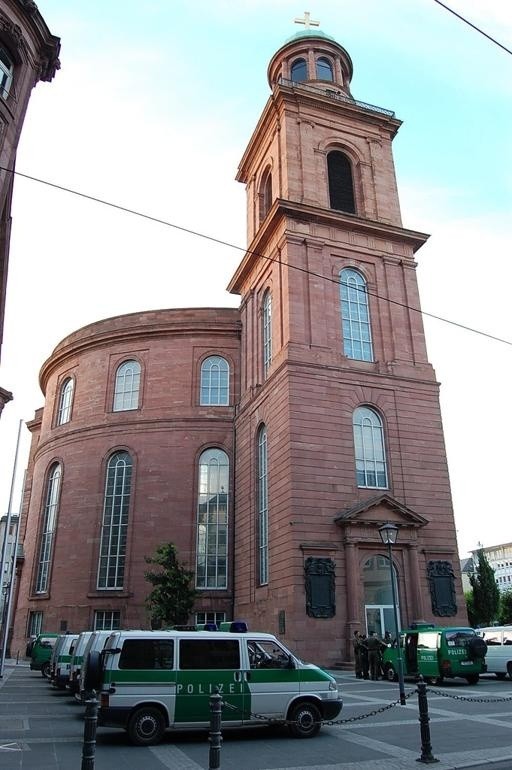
352 631 394 681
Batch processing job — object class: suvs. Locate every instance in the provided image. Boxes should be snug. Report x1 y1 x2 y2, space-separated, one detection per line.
381 621 512 686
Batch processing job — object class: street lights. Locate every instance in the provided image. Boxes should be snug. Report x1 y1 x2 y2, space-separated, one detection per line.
377 519 406 705
